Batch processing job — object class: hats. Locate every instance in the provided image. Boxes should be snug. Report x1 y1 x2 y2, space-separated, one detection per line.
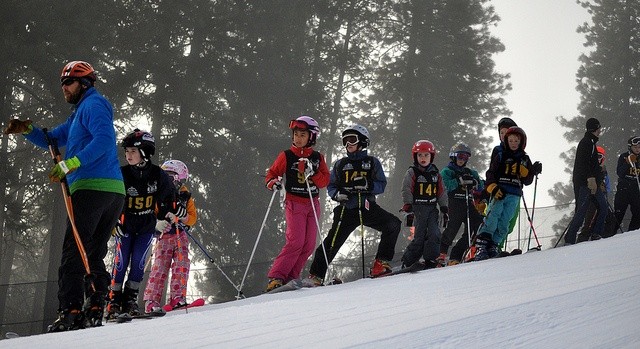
586 117 600 132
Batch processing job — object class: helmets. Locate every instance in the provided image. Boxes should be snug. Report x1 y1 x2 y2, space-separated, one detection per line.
122 128 155 162
597 146 606 164
504 126 526 149
628 136 640 151
448 142 471 164
296 116 319 148
60 60 96 81
498 117 517 133
412 139 436 163
160 160 189 183
342 124 371 149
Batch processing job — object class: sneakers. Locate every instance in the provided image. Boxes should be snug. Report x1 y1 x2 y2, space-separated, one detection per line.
473 238 490 261
52 309 85 332
144 300 163 314
401 262 414 269
122 288 140 316
370 258 391 276
591 233 601 240
436 253 447 267
447 259 460 266
265 277 284 293
168 294 190 310
425 260 446 268
308 273 322 287
104 288 122 322
487 240 499 258
84 291 107 328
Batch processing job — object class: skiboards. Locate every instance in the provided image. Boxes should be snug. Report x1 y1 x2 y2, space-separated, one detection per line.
326 261 421 286
525 246 540 254
496 249 522 258
162 298 205 312
6 332 19 339
266 278 315 294
107 306 167 323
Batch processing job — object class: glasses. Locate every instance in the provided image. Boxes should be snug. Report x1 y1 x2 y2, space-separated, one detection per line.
597 153 605 160
342 134 360 147
61 78 81 86
289 120 320 132
169 174 179 182
632 137 640 145
448 152 470 161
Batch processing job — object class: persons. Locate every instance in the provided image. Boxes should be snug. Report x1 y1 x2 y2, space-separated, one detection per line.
401 140 448 269
565 118 606 244
490 118 543 253
614 136 640 234
102 128 176 323
474 126 534 261
308 124 402 286
577 146 610 243
143 159 197 314
1 61 126 330
437 141 485 266
264 115 330 292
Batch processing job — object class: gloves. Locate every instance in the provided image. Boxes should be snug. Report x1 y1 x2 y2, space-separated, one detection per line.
4 119 33 135
172 199 188 217
459 174 473 185
111 219 124 238
272 182 282 190
49 156 82 183
354 176 369 190
335 190 349 202
471 179 480 189
486 182 508 200
291 158 315 176
512 163 529 177
402 204 413 213
530 161 542 175
440 206 448 213
